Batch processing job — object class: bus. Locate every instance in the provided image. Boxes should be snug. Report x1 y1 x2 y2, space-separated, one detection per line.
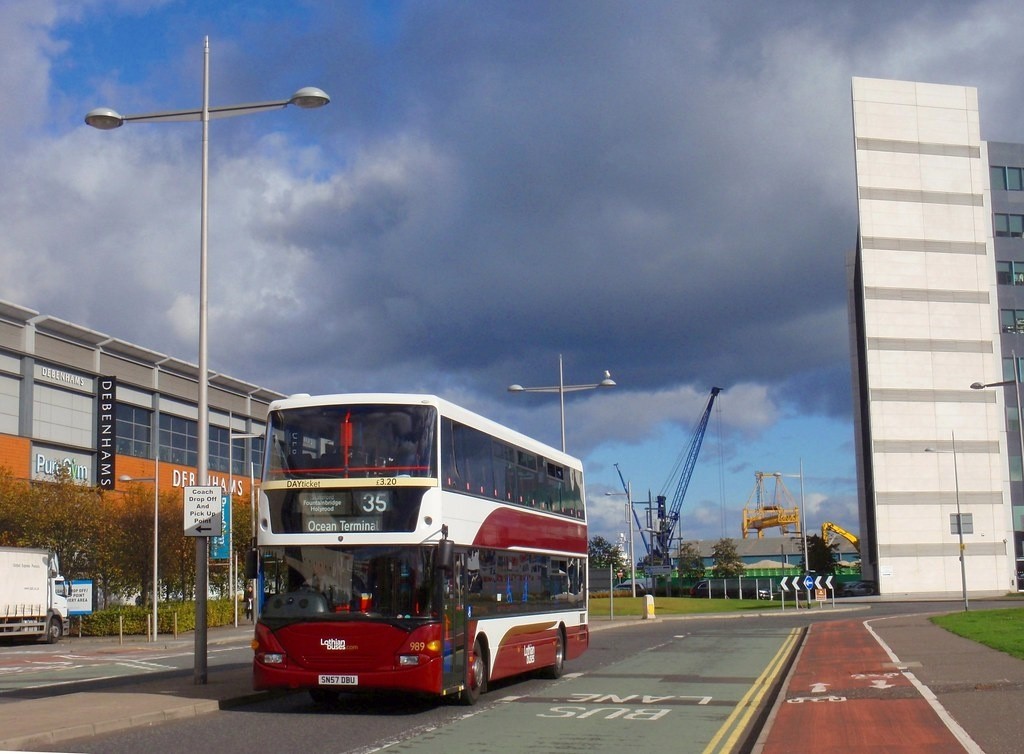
249 392 589 707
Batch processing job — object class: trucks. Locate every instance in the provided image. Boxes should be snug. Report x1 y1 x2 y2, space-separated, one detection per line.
0 545 72 646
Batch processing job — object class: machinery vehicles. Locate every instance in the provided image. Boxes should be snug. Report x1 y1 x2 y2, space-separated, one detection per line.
819 519 862 560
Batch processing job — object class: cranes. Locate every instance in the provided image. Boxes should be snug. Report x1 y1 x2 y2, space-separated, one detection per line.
613 381 722 599
